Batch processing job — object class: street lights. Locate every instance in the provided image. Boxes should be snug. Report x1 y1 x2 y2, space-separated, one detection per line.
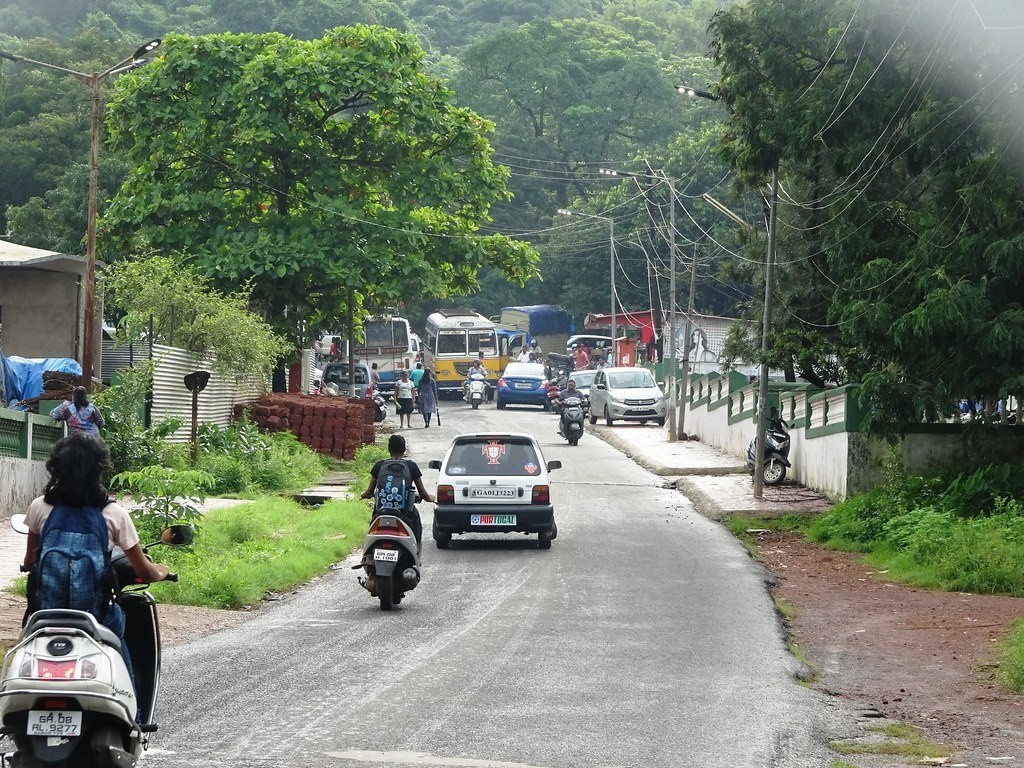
597 167 679 444
556 207 618 369
674 84 779 500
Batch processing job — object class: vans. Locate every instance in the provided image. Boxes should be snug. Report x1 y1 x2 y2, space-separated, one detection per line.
566 334 612 365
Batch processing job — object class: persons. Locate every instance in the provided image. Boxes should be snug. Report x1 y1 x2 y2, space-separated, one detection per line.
569 340 590 371
49 386 105 441
555 381 584 424
161 525 185 545
529 339 542 354
370 362 380 390
328 336 340 362
394 370 415 428
749 375 757 384
477 351 485 367
416 369 438 427
463 359 491 403
313 340 324 367
518 346 532 362
21 432 170 726
527 353 537 363
361 435 435 545
689 328 708 361
596 357 611 369
409 363 425 388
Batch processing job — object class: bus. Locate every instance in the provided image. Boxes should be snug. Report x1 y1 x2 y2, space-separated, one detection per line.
296 306 529 401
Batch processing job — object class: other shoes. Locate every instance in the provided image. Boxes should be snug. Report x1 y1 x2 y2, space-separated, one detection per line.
408 425 411 428
400 426 403 428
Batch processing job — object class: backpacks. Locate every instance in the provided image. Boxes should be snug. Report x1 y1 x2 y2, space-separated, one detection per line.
373 459 411 513
26 504 108 624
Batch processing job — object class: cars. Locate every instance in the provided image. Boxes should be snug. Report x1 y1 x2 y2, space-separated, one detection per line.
588 367 667 427
313 362 375 399
429 431 563 550
568 369 598 415
496 362 551 412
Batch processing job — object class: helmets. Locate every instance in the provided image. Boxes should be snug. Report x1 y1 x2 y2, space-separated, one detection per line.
531 339 538 348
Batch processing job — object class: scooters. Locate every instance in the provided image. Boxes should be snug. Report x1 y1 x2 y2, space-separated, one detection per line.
465 370 487 410
0 512 196 767
554 394 590 446
351 485 439 612
744 403 796 488
